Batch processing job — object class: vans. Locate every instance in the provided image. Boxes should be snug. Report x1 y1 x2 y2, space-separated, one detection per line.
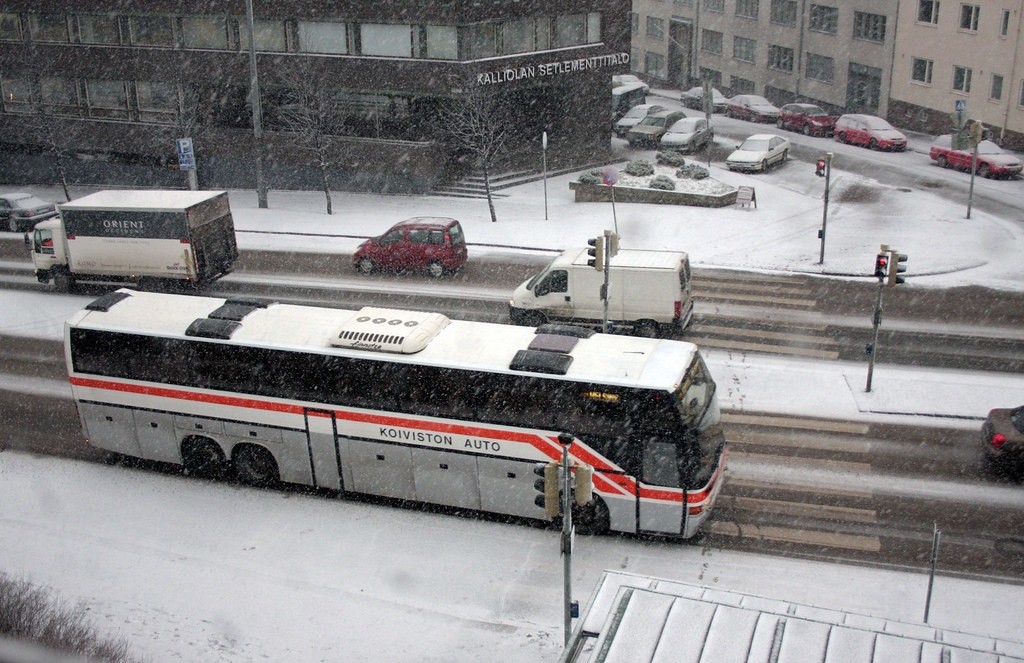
507 246 696 340
354 216 469 280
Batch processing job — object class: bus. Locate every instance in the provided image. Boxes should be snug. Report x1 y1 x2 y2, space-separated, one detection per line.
63 286 729 542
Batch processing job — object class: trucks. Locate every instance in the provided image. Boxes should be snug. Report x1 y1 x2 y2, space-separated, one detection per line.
23 188 241 293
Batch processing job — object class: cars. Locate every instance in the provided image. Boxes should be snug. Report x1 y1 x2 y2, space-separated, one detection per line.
726 133 793 174
613 103 662 135
837 113 907 152
661 117 715 154
612 74 650 96
0 192 60 234
627 109 686 146
779 103 836 137
929 132 1023 181
982 404 1024 483
681 87 727 113
725 94 780 124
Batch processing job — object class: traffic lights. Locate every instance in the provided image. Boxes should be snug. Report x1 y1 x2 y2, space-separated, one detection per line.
612 233 622 256
534 461 560 516
874 253 890 280
889 248 908 286
816 157 826 177
587 237 604 272
